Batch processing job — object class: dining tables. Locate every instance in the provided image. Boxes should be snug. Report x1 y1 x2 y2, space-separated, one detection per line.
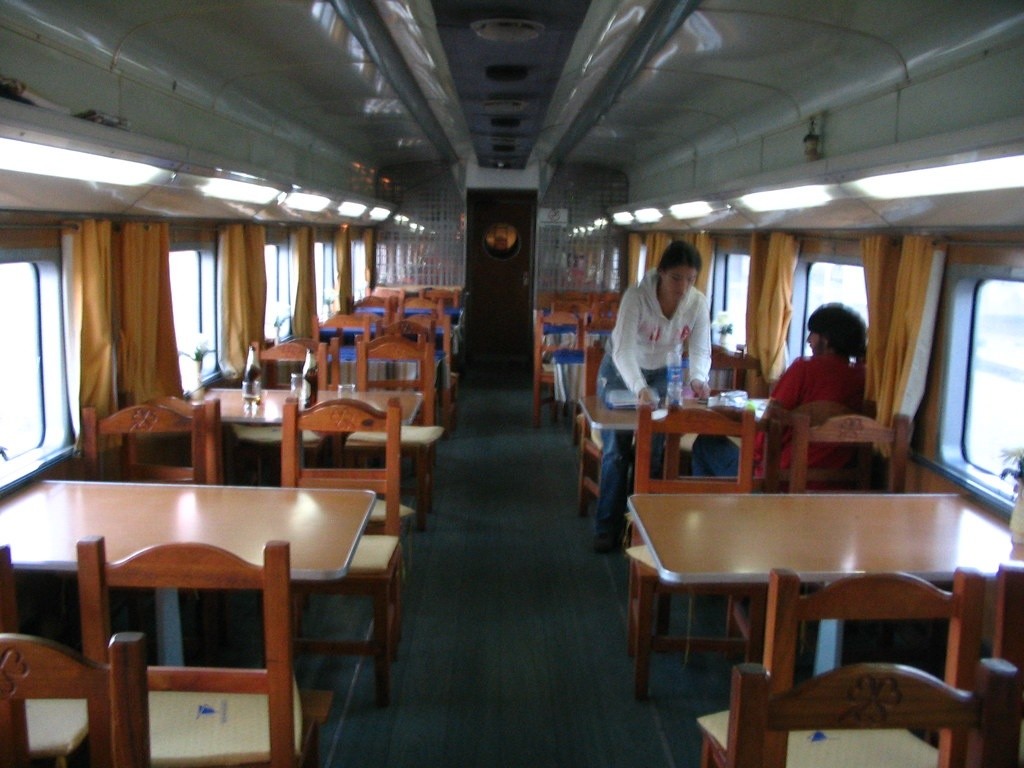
577 395 785 434
205 387 424 487
1 479 378 665
282 402 404 708
353 306 465 326
628 492 1024 678
553 348 732 403
272 346 448 385
542 326 613 372
317 325 457 352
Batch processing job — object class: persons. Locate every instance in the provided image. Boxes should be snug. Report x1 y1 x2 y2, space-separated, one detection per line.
591 238 713 554
688 302 880 497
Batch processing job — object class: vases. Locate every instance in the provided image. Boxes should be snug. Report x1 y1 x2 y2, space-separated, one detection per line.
275 327 282 346
196 360 203 382
1009 495 1024 543
720 332 728 344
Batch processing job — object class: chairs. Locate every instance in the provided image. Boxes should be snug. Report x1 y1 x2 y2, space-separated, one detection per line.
124 287 467 536
80 402 208 666
790 410 910 657
534 290 745 443
577 345 606 519
0 632 152 768
698 566 986 768
662 403 768 494
727 657 1020 768
766 398 877 493
77 532 336 768
626 403 771 703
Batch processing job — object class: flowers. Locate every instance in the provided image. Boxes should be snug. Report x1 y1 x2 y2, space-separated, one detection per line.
1000 444 1024 497
272 299 291 327
712 309 733 333
179 344 214 361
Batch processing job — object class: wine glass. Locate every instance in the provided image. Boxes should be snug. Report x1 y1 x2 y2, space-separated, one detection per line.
242 381 259 410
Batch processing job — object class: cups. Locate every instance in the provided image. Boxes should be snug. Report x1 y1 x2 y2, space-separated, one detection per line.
290 373 302 399
338 384 355 400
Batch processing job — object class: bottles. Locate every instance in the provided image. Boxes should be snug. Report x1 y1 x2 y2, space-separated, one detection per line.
301 348 318 409
665 349 682 405
242 347 260 411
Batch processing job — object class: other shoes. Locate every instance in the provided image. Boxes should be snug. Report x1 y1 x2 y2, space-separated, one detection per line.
593 531 618 554
